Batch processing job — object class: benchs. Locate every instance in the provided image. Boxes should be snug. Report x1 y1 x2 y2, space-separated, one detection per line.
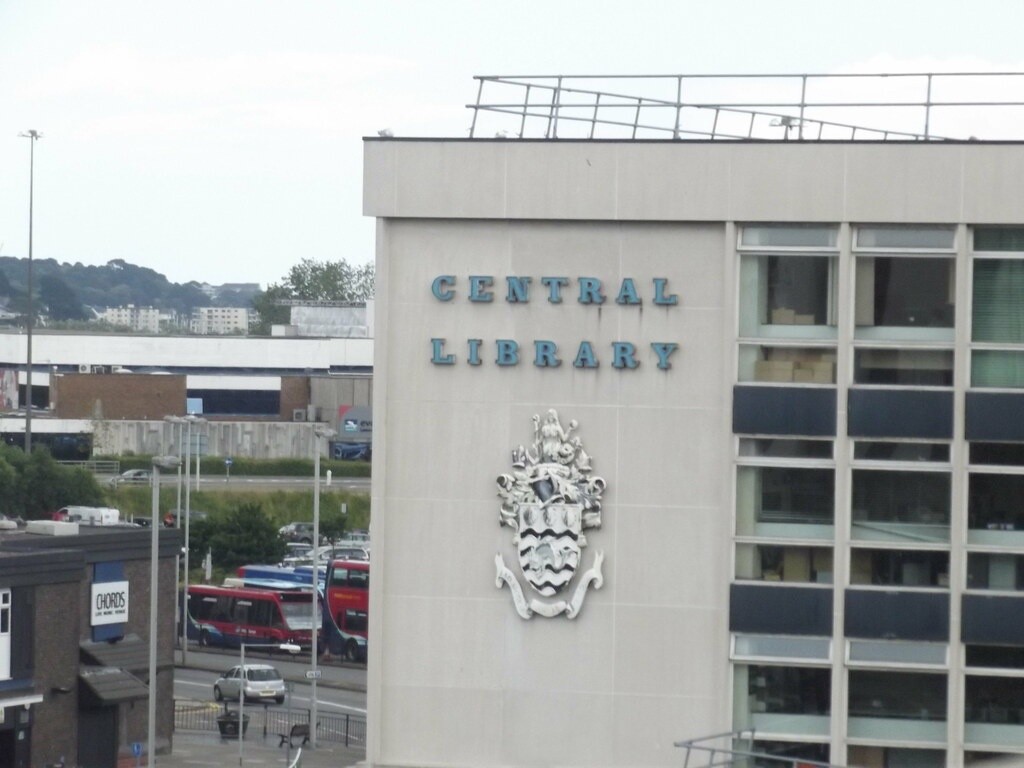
279 722 321 748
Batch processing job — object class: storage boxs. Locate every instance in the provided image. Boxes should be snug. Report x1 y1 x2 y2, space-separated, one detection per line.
763 546 834 584
754 347 834 384
848 746 886 768
772 309 814 325
849 549 948 585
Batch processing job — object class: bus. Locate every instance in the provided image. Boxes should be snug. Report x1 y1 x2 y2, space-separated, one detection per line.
224 555 370 664
177 583 330 659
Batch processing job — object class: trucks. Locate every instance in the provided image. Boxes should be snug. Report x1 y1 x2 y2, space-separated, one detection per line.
52 505 121 528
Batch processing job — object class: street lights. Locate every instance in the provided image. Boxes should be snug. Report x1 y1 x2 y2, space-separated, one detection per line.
18 128 43 451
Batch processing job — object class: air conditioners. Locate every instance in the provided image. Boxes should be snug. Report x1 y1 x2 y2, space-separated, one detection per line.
293 409 306 421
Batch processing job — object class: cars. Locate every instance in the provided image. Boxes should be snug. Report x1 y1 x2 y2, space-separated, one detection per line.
126 508 370 584
111 468 154 485
212 662 287 705
